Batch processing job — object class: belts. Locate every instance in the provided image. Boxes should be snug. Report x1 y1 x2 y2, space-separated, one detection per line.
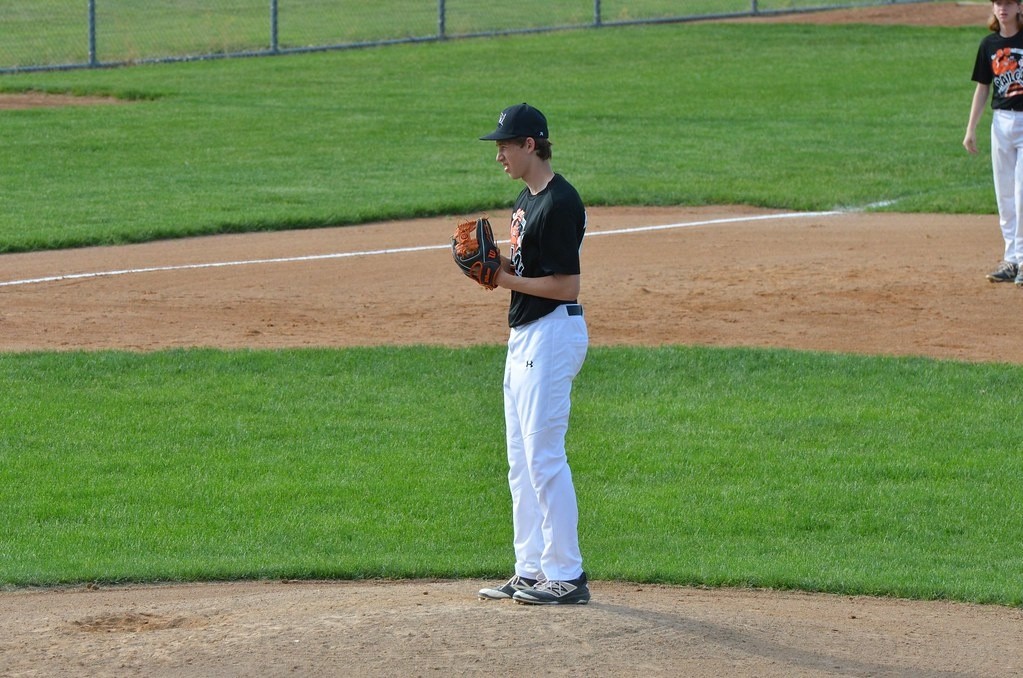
566 305 583 317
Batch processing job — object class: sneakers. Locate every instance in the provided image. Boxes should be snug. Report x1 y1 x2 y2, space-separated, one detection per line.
985 261 1019 280
1014 262 1023 286
478 570 548 599
513 570 592 605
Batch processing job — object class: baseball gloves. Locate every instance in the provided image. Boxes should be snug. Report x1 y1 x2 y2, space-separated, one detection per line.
450 217 502 291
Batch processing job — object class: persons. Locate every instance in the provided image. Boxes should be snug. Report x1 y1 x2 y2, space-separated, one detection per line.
963 0 1023 286
452 103 590 605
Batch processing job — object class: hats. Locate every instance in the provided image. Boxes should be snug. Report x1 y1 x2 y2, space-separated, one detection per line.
479 102 550 140
